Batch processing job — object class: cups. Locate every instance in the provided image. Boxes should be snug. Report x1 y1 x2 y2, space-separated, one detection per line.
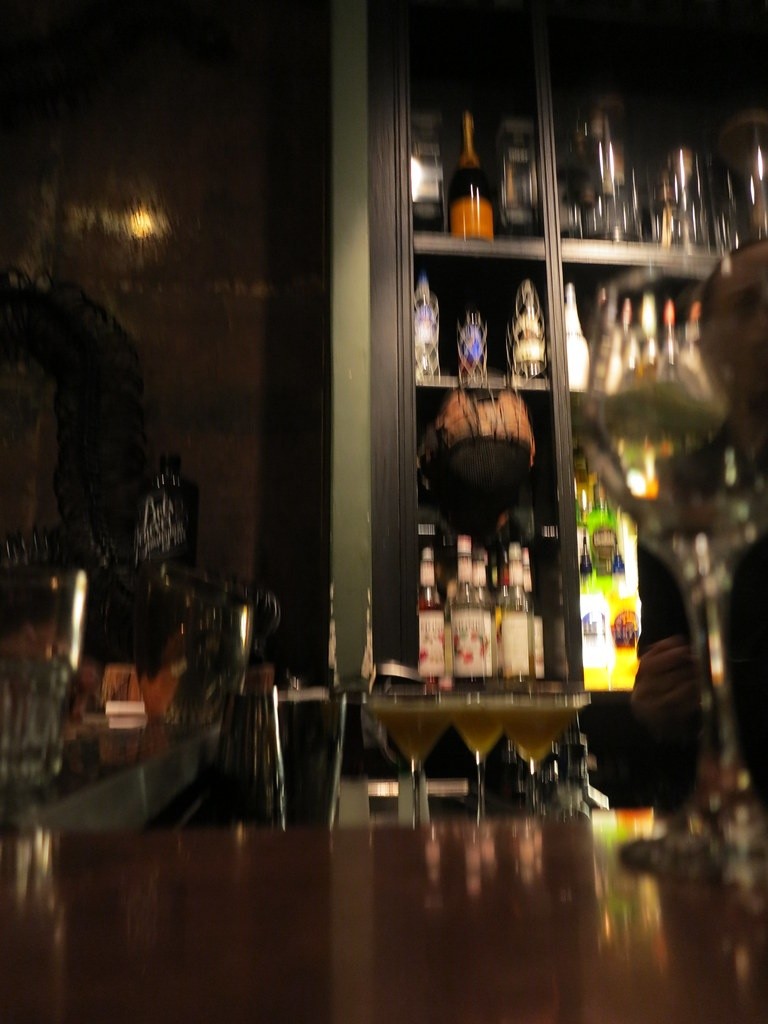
500 121 537 239
0 659 64 789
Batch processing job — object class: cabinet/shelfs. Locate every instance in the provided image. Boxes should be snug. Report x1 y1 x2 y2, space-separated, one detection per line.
329 0 768 824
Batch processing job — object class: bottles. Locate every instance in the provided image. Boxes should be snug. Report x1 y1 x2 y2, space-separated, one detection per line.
418 534 545 700
577 506 639 691
447 110 494 240
412 272 545 380
563 280 702 395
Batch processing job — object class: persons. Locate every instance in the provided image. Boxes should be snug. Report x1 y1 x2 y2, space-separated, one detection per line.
631 242 768 809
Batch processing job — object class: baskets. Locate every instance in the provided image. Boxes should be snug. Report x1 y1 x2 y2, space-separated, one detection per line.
443 435 530 503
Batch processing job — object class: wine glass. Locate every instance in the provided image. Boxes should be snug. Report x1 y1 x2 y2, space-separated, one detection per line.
579 390 764 883
368 697 594 827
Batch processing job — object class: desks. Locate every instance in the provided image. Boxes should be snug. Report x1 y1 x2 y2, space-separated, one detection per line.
0 799 768 1024
